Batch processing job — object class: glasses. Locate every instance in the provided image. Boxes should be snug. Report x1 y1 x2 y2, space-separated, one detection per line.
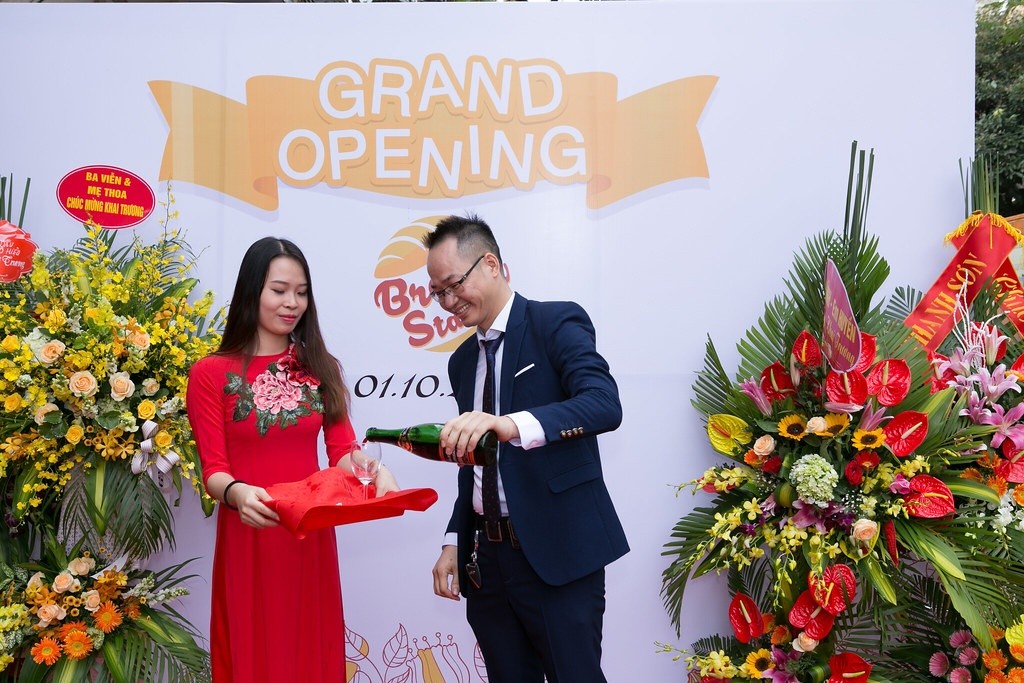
430 254 483 300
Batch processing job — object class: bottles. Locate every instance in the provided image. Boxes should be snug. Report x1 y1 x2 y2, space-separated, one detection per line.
366 422 499 467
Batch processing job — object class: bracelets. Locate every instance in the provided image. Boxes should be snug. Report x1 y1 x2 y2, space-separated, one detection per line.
223 480 246 511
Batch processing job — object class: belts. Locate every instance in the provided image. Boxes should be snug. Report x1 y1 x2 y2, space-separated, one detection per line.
475 517 511 543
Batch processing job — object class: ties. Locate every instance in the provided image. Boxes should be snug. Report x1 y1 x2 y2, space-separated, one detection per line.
480 332 505 526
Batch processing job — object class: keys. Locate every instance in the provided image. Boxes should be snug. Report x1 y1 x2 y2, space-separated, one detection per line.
466 562 482 591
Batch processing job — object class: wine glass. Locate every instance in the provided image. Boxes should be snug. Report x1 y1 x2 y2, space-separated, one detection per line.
351 440 383 500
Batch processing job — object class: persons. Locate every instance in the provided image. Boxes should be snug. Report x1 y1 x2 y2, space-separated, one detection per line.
421 215 631 683
184 237 403 683
